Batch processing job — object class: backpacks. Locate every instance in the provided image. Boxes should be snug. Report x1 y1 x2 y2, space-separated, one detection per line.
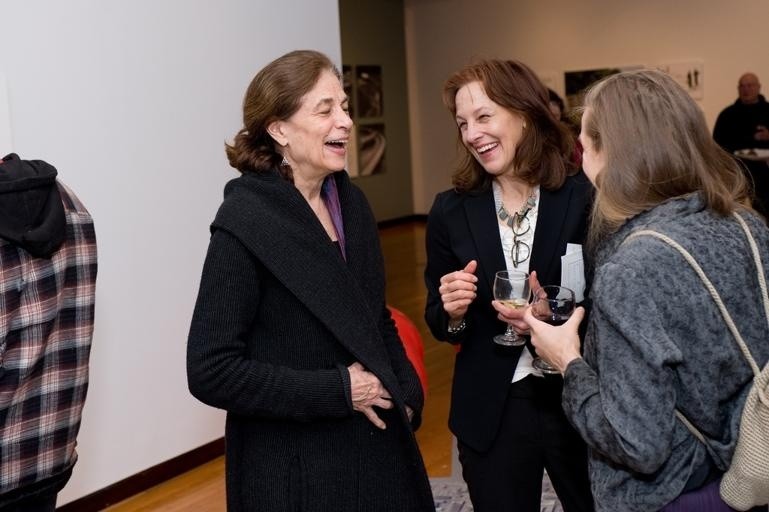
620 210 769 511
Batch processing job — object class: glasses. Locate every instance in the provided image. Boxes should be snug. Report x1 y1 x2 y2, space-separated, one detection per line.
511 208 532 268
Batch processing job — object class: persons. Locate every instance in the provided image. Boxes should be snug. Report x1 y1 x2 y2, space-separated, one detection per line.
186 51 436 512
527 70 769 512
423 58 599 512
544 88 566 122
713 73 769 235
0 153 98 511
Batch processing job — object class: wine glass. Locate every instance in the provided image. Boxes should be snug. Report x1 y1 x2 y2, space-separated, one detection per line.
531 286 576 378
493 272 532 346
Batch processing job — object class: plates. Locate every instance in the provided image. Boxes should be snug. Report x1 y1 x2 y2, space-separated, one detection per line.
734 148 768 160
